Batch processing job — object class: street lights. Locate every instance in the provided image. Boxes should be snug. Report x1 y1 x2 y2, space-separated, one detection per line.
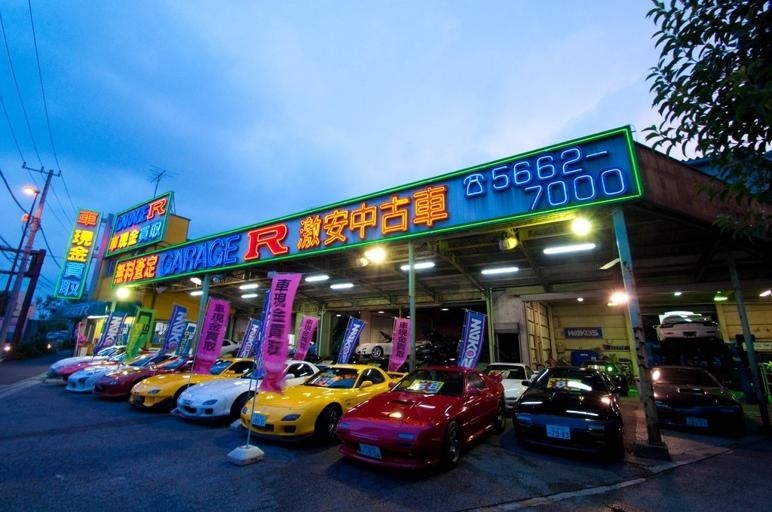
713 273 772 430
0 185 41 359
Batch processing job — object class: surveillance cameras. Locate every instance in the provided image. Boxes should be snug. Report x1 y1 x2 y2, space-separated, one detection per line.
599 258 620 272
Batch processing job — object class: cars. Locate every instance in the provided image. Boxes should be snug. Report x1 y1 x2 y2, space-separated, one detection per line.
240 366 410 445
45 337 335 424
583 360 629 397
335 364 507 471
354 331 433 362
512 366 626 468
483 361 539 410
648 312 734 428
